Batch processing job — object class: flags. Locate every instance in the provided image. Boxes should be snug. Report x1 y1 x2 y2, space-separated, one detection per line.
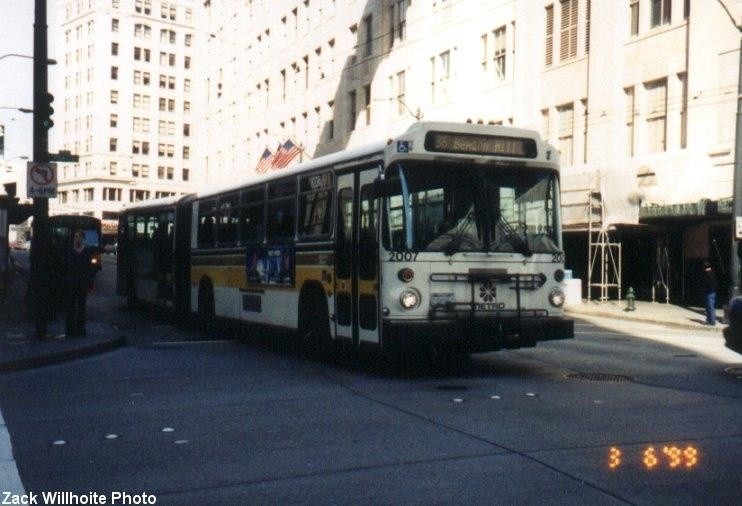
270 144 282 172
273 139 301 169
256 148 273 176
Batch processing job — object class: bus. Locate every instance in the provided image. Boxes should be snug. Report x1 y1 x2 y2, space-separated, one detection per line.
117 130 577 375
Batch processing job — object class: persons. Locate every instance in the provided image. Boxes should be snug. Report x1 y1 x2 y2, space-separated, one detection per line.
32 231 74 342
274 249 290 287
700 261 717 327
64 228 98 337
245 248 263 284
